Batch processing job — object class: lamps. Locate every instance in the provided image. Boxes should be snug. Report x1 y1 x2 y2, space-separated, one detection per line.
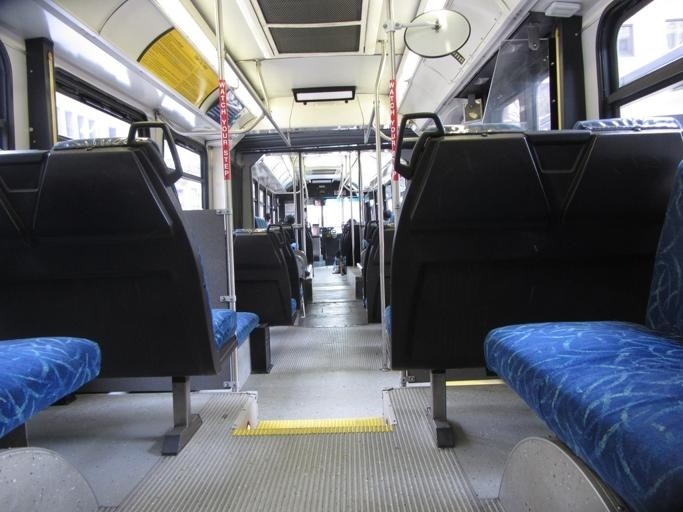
292 86 355 102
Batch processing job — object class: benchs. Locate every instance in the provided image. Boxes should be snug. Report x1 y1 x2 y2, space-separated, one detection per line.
338 217 403 326
232 223 314 325
3 122 260 456
383 110 671 450
1 338 102 510
482 162 683 512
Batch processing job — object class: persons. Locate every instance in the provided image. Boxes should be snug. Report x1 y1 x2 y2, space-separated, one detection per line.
264 213 271 226
361 208 393 253
281 215 295 244
331 219 360 274
